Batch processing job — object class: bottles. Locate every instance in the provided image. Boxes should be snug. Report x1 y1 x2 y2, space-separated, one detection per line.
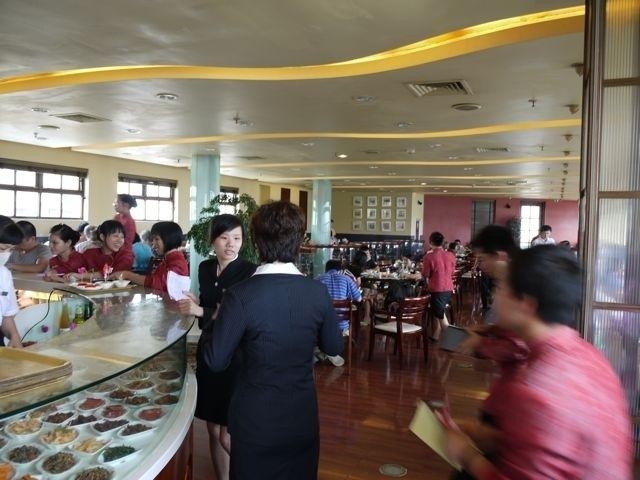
57 300 93 335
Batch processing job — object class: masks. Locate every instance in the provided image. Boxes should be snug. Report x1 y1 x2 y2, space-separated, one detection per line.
0 252 12 265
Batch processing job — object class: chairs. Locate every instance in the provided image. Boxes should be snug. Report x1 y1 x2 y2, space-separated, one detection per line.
321 239 492 369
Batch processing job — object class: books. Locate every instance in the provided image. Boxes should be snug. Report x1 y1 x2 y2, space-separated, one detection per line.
408 396 486 474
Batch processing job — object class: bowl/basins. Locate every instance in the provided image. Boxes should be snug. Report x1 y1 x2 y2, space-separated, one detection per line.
97 279 131 290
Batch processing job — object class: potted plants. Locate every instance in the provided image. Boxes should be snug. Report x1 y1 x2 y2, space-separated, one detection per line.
182 192 267 265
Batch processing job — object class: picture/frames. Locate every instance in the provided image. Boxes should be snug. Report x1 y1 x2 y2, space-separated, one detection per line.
352 196 406 232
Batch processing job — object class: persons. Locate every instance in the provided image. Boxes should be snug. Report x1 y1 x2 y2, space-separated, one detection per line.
448 239 463 261
0 213 26 352
75 195 154 274
422 232 455 340
110 222 188 291
433 243 636 478
5 221 52 275
456 224 532 397
393 253 425 287
63 220 135 280
314 258 361 367
45 222 86 284
349 250 378 326
533 225 555 246
201 201 346 480
176 215 259 480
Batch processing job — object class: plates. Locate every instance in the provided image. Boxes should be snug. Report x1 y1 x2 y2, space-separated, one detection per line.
70 282 103 291
0 359 184 480
